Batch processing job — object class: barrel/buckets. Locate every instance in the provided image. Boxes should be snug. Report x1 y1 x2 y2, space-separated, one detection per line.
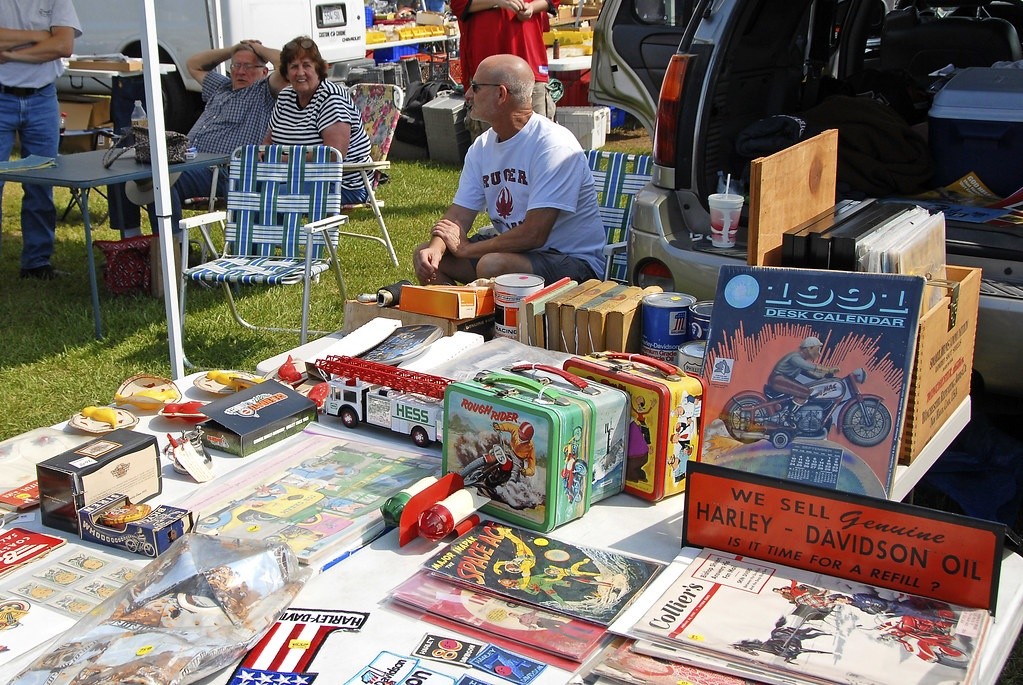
640 291 715 377
493 272 545 340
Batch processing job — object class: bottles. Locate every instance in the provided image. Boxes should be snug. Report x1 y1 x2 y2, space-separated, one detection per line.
130 100 148 128
59 112 67 148
553 38 560 59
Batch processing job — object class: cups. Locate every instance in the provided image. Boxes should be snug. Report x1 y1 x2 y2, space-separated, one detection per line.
708 193 744 249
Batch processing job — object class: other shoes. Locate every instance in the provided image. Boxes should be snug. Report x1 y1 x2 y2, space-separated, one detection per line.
20 265 71 280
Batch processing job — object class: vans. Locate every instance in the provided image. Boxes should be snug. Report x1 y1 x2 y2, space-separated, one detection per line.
56 0 456 135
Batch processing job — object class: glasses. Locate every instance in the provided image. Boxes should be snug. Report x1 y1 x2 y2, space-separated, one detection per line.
285 40 318 50
230 62 266 72
470 78 513 94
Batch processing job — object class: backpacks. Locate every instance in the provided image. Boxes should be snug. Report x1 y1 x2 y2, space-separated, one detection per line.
395 81 455 147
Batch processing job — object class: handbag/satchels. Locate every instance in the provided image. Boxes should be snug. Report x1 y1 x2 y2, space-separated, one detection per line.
101 124 193 168
94 234 159 299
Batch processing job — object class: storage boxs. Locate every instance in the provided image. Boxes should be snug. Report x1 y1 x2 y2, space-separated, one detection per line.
554 104 626 150
896 265 983 468
195 379 317 457
421 94 470 162
364 6 462 85
400 284 495 321
69 61 143 71
928 67 1023 200
56 94 113 154
36 428 193 560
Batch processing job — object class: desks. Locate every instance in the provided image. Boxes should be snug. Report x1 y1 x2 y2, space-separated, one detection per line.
366 34 460 81
547 55 592 86
62 63 176 134
0 148 230 343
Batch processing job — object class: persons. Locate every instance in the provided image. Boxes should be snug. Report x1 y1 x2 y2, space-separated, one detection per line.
226 37 376 286
0 0 84 279
418 0 449 14
413 54 607 285
450 0 563 141
104 39 288 258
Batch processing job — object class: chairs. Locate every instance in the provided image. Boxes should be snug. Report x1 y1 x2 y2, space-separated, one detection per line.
61 83 404 375
583 148 654 286
880 0 1022 76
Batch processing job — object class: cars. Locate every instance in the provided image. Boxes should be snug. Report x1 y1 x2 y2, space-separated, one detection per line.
585 1 1023 406
